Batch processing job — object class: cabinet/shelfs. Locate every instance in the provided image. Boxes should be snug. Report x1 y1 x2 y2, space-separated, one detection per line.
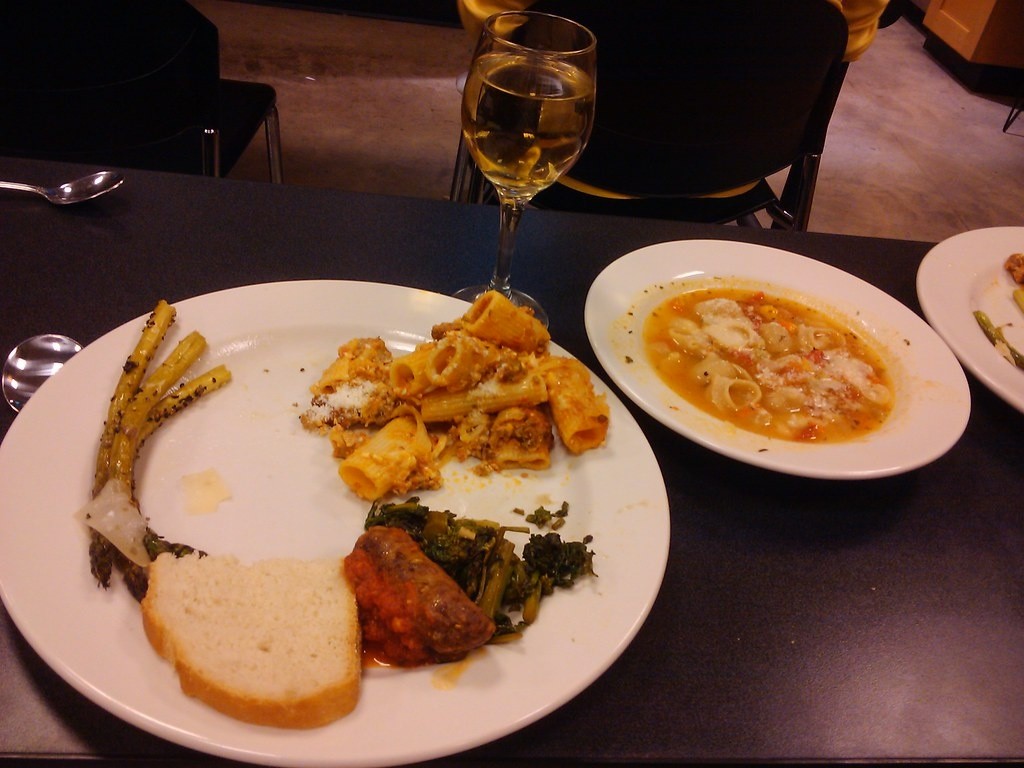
909 0 1024 67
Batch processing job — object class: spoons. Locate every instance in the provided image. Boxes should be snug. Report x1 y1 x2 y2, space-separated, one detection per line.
0 171 124 204
2 333 83 413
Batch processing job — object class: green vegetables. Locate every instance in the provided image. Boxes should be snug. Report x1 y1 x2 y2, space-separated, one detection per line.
363 494 598 643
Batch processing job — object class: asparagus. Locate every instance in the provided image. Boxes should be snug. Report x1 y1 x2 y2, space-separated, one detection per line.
85 301 233 604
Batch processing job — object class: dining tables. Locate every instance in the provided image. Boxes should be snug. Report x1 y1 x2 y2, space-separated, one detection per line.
0 156 1024 768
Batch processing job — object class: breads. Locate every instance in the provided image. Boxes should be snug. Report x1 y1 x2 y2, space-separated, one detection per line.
141 550 361 730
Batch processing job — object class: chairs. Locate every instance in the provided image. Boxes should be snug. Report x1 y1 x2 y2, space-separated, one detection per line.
449 0 849 231
0 0 282 188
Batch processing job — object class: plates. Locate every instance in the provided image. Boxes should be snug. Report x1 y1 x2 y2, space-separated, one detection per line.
916 227 1024 414
584 240 970 480
1 280 670 764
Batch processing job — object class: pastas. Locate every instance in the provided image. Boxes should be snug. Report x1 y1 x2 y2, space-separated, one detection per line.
309 289 610 502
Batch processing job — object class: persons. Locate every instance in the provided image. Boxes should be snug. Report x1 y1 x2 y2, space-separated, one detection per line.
455 0 891 201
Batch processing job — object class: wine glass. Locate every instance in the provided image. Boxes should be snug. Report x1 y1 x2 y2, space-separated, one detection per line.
451 11 597 333
